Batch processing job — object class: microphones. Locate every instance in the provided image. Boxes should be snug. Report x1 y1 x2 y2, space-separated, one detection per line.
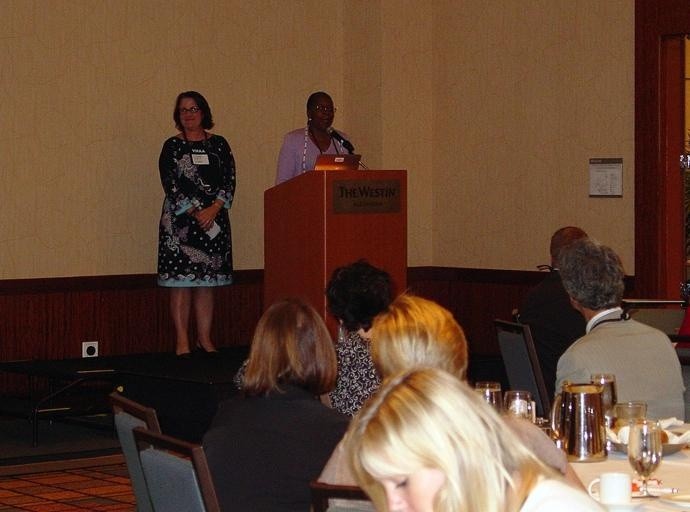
326 126 354 151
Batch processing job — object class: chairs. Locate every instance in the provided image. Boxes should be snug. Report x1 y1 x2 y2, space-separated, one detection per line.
491 318 551 419
307 478 379 511
620 297 686 336
107 391 161 511
130 424 221 511
667 332 689 423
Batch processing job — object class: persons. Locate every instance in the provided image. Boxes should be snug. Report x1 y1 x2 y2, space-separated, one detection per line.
317 293 590 511
343 366 603 512
157 91 236 361
200 296 350 511
554 239 686 428
276 90 354 185
318 261 392 421
522 226 592 406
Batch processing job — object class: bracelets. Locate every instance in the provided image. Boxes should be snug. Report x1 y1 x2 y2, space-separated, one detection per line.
188 207 198 218
211 200 223 210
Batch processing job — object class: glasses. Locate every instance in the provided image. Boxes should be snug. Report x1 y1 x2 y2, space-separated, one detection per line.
312 104 336 112
177 106 200 113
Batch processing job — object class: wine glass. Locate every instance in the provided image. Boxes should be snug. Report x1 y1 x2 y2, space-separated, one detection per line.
590 373 616 427
627 420 664 499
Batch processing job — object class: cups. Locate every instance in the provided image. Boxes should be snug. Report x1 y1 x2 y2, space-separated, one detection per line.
588 471 632 507
617 401 648 455
474 380 502 413
504 390 534 423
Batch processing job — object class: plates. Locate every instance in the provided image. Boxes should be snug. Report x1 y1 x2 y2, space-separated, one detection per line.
610 433 687 459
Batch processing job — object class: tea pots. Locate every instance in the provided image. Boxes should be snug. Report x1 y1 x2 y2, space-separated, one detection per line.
550 381 608 464
